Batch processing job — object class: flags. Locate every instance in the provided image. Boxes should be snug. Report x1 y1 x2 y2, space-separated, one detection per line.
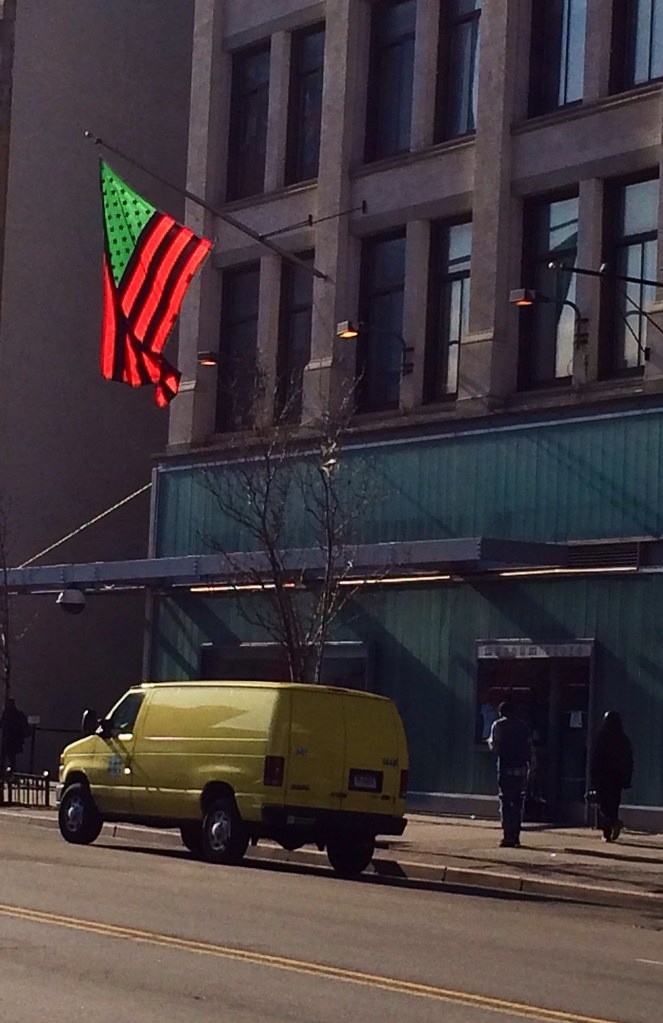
96 158 211 408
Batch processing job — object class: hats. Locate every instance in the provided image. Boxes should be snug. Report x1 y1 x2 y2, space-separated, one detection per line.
499 701 511 712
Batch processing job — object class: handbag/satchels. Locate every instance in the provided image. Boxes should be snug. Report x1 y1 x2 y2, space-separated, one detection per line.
17 711 31 737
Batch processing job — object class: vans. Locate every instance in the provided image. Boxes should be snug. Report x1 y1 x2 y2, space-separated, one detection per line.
56 682 408 874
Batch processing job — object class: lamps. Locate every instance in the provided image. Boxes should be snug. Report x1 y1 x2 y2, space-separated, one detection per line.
509 288 590 350
197 350 217 370
337 320 415 378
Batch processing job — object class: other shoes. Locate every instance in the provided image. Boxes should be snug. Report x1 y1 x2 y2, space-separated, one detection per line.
613 819 623 840
500 839 520 847
603 826 612 841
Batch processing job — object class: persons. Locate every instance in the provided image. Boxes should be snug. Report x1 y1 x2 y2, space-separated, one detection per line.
587 709 633 842
487 700 536 848
0 698 32 772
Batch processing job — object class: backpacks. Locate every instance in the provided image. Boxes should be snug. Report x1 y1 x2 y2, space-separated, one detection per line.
493 717 531 766
